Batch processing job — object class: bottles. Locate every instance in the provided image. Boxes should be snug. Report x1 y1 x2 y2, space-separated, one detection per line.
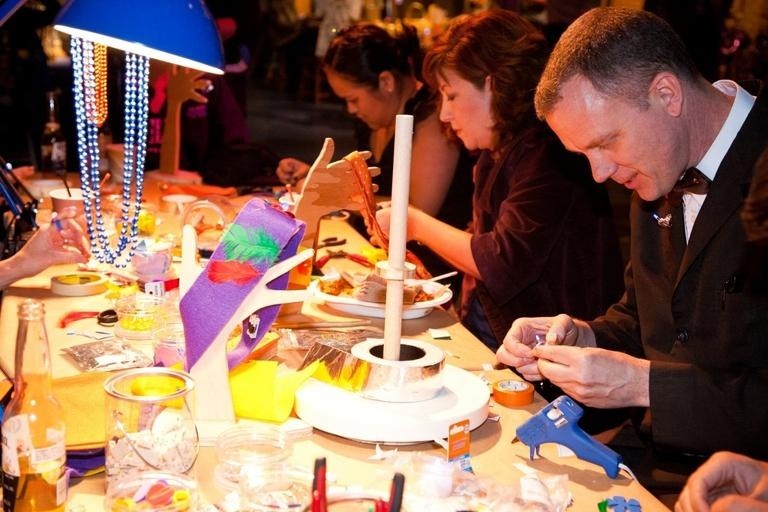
41 92 67 178
1 301 69 512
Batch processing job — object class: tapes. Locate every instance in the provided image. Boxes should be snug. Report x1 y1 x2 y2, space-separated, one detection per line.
49 272 108 297
493 380 535 407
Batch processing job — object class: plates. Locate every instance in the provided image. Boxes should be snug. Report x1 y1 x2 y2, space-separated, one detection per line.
310 270 456 321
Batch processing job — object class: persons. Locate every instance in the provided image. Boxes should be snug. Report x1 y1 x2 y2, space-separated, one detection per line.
217 19 253 149
496 7 767 503
364 9 624 346
278 25 476 297
0 0 153 170
279 27 305 99
1 205 93 287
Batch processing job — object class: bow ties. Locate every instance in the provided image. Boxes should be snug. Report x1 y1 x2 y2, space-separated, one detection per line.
664 165 712 204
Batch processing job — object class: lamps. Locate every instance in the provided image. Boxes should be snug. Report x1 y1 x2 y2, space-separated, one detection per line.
53 1 228 80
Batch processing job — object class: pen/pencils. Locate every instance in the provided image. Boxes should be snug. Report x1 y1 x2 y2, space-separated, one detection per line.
110 268 150 293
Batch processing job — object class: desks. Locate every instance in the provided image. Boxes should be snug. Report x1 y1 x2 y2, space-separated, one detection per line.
2 177 674 512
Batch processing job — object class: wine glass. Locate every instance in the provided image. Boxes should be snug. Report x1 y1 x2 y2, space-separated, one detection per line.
105 367 200 478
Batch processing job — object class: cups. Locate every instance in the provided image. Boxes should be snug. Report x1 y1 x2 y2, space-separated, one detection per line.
109 143 137 184
134 242 171 276
212 421 295 507
154 331 186 369
47 188 96 248
161 193 198 217
113 297 168 331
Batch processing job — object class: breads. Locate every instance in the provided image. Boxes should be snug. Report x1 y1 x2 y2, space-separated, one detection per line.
358 274 423 306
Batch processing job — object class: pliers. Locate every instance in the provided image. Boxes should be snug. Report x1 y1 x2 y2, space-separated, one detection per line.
317 237 346 248
316 250 372 269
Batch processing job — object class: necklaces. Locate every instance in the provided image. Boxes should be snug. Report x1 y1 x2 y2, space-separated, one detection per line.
68 35 149 269
